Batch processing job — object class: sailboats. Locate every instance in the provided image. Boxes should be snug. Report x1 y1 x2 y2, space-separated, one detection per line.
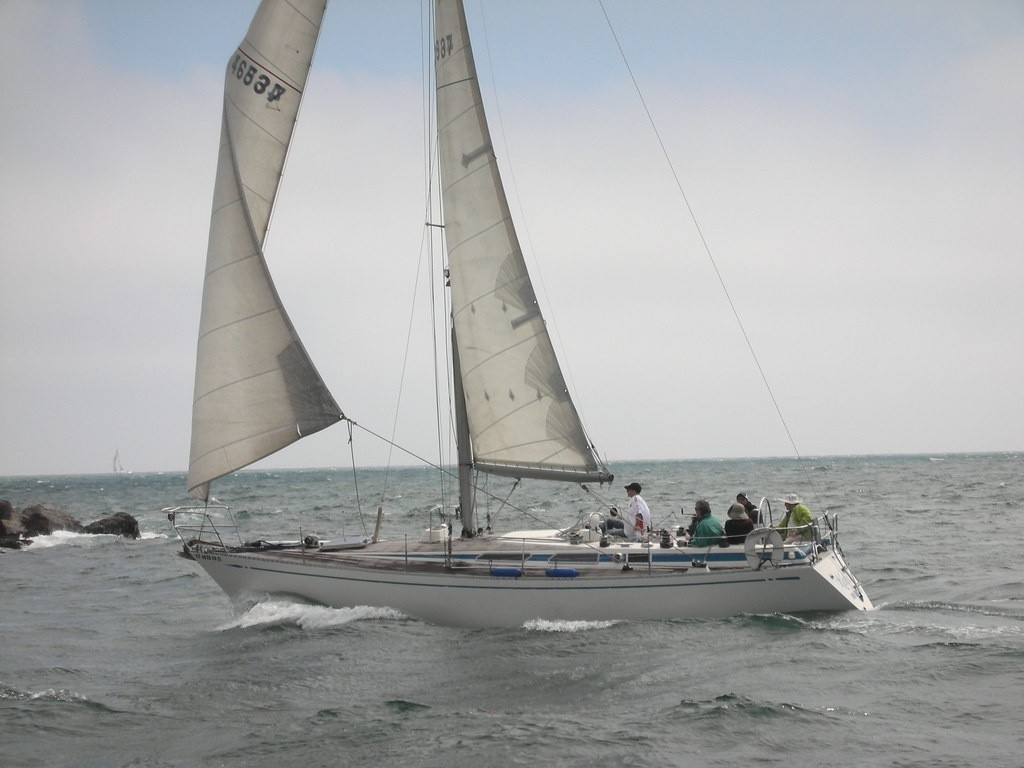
159 1 874 630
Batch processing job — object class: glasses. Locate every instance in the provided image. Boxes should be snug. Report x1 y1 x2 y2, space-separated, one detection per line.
694 507 701 511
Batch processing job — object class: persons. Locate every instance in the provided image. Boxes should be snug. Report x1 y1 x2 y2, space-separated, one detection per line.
685 492 762 547
599 482 649 538
773 493 813 542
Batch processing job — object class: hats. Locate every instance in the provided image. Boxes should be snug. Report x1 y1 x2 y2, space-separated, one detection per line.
737 493 749 501
778 493 803 504
624 483 642 492
727 503 749 520
